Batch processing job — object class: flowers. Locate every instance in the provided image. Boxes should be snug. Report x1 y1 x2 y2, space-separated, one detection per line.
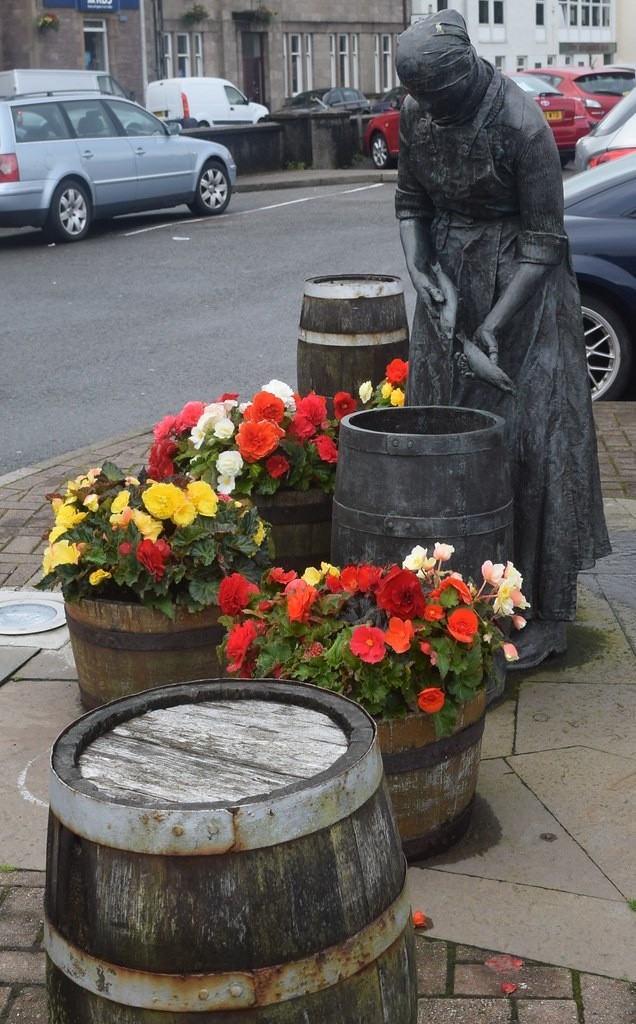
181 3 208 27
252 5 272 26
211 540 529 743
144 352 410 497
36 11 60 35
36 464 270 616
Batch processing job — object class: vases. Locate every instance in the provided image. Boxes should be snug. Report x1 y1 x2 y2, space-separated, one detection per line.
60 590 235 716
330 672 489 871
138 467 335 579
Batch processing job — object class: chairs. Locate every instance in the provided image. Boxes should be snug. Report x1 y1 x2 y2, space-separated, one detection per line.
600 77 616 89
77 116 105 138
23 128 49 142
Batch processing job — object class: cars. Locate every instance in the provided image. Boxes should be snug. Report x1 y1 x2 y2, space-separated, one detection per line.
556 151 636 401
364 72 590 169
281 87 370 116
575 86 636 175
521 62 636 126
371 85 406 114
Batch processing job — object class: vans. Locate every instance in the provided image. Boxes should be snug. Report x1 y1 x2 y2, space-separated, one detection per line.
0 68 147 138
145 77 270 130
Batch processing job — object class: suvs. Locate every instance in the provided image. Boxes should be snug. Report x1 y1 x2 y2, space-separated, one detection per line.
0 90 237 245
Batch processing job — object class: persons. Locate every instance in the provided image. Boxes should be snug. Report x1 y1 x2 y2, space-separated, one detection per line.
395 9 612 668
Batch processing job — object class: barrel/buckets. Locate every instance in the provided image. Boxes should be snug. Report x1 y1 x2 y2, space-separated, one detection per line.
41 678 418 1024
298 273 409 413
329 404 514 711
